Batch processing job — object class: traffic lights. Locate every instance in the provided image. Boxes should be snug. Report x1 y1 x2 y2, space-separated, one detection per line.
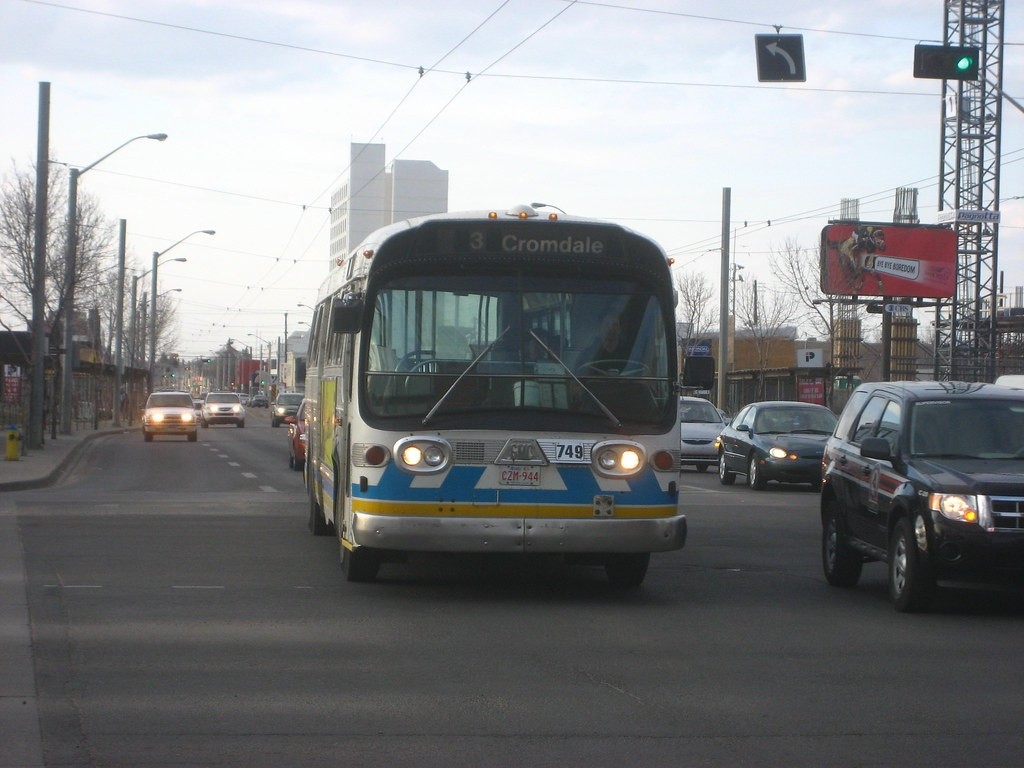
261 381 265 385
201 358 211 363
912 43 981 80
164 374 175 377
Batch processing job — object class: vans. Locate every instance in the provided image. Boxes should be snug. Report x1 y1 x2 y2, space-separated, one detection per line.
995 374 1024 414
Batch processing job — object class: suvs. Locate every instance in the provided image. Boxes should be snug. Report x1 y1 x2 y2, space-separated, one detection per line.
820 379 1024 609
200 391 245 427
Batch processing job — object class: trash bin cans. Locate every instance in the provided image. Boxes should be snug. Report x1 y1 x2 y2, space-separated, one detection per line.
4 423 22 461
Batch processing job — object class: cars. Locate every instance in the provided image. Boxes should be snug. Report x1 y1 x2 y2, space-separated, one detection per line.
251 395 268 408
141 390 197 441
679 395 727 472
715 399 839 487
283 398 308 469
238 393 250 405
270 393 305 427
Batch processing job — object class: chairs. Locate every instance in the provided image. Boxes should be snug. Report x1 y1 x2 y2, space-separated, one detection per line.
367 340 399 400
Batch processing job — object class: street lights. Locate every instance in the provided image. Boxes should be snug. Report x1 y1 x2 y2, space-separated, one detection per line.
297 322 310 326
148 229 216 393
133 288 182 363
531 202 565 213
62 131 167 431
296 302 314 309
231 339 252 399
129 257 187 424
247 333 272 400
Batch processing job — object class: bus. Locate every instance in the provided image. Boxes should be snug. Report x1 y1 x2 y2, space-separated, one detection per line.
303 206 686 584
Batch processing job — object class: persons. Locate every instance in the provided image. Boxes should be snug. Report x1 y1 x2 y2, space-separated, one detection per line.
522 328 569 363
576 308 648 378
120 387 129 421
